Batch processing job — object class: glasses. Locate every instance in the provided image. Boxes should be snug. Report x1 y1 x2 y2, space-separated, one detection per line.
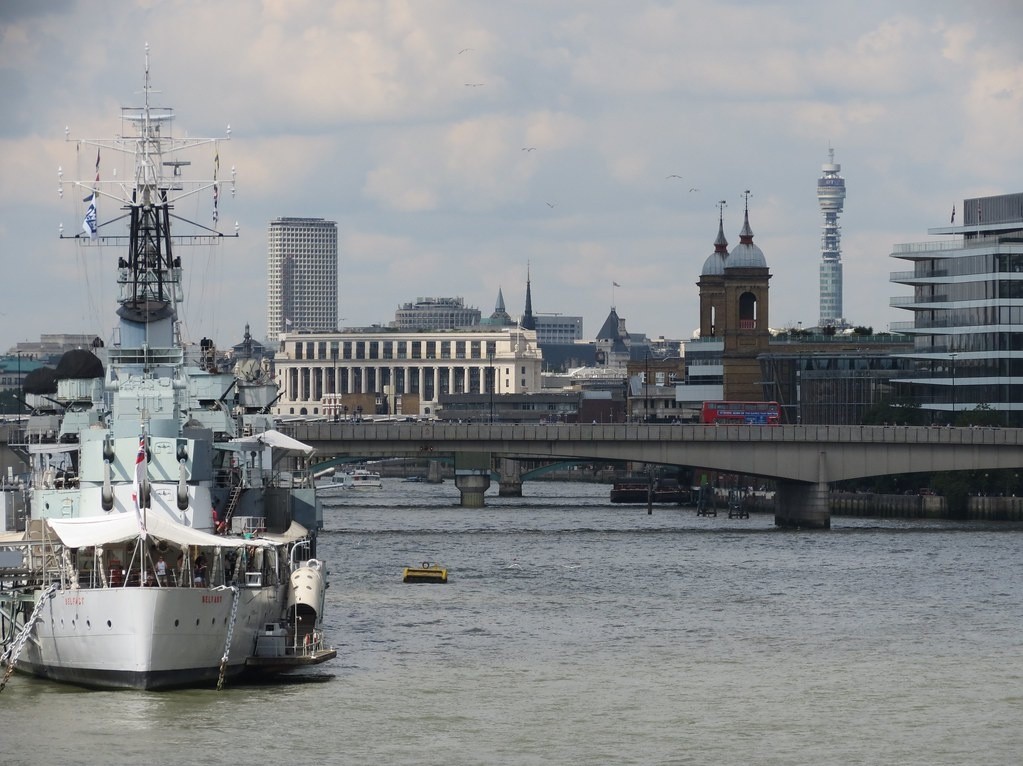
160 558 163 559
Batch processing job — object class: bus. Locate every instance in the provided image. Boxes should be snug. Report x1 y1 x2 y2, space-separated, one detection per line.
702 401 781 426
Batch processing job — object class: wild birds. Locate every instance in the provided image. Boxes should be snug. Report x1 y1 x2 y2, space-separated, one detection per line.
950 202 956 223
521 147 536 152
465 83 485 87
666 175 683 179
545 202 561 208
689 187 698 193
459 49 473 54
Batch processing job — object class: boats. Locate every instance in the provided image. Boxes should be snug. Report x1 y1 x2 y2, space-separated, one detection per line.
330 469 381 488
402 562 447 584
1 38 339 693
610 476 691 503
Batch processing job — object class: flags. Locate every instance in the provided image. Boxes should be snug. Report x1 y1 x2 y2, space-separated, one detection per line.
212 150 220 226
82 148 100 242
133 431 148 463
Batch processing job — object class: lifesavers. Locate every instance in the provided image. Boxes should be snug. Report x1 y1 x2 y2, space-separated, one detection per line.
422 562 429 569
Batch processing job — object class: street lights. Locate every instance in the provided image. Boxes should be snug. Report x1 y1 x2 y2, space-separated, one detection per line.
799 354 803 426
949 354 958 430
17 350 22 429
490 351 494 427
646 353 648 426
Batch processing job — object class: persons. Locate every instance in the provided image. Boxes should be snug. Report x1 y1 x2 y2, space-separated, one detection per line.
225 554 231 570
247 557 254 572
194 550 207 587
155 557 168 587
177 555 184 575
